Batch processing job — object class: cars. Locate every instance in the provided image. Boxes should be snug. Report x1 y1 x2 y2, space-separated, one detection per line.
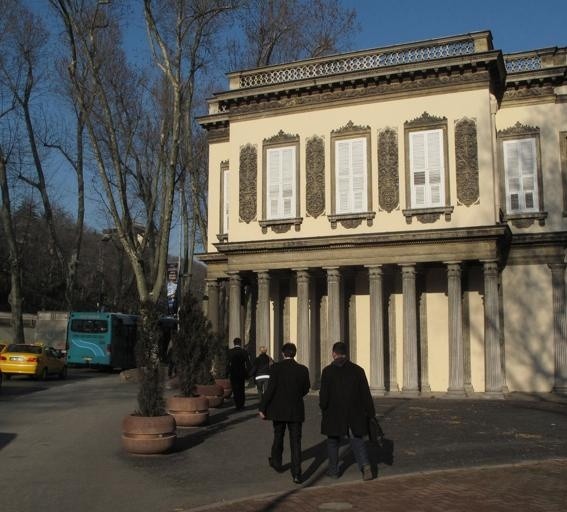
47 342 66 360
0 340 67 380
0 343 9 354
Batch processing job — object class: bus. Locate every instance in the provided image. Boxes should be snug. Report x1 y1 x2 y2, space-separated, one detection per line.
65 310 139 372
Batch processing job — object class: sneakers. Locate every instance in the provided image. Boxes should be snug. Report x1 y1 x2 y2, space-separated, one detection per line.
268 457 282 473
293 476 303 483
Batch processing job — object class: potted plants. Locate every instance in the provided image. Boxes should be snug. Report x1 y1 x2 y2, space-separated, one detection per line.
114 299 181 453
168 302 213 425
208 331 232 396
184 297 224 407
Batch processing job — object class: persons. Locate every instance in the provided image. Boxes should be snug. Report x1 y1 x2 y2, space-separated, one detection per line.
319 342 378 481
224 338 249 410
260 342 311 484
250 346 277 401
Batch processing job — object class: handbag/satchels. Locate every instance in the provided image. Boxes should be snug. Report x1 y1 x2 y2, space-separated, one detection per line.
367 419 384 449
